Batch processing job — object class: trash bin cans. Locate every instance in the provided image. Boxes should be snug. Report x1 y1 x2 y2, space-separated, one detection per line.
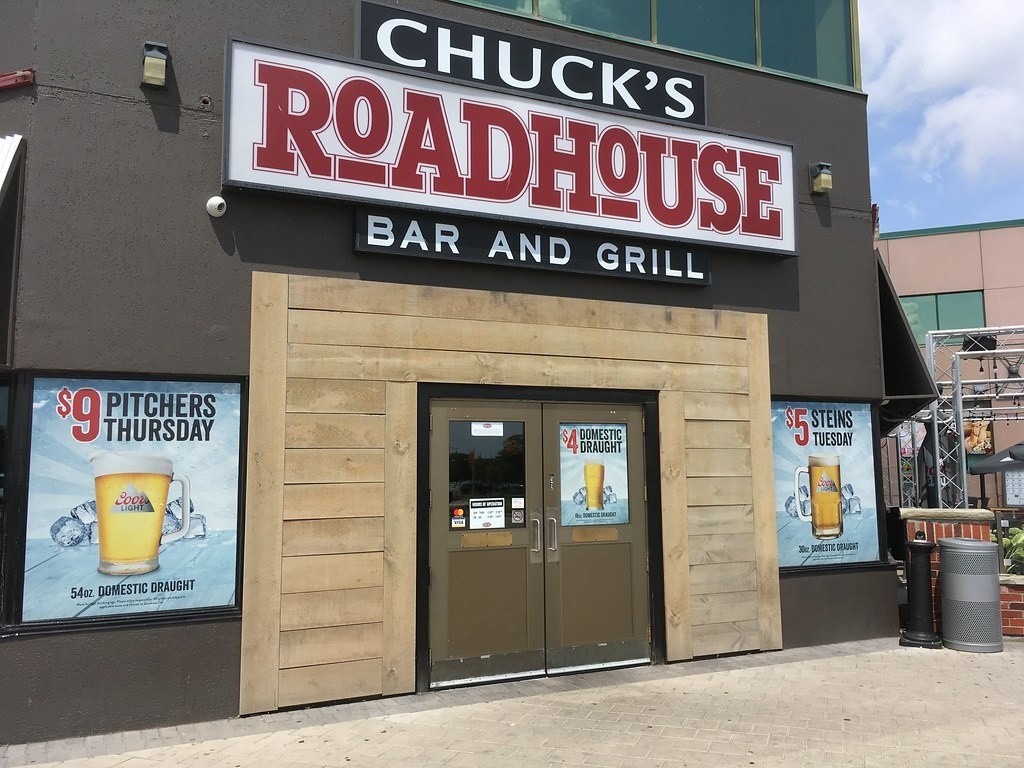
934 536 1005 652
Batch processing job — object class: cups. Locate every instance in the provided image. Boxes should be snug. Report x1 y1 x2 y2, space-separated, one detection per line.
794 454 843 540
91 456 190 576
583 457 605 511
